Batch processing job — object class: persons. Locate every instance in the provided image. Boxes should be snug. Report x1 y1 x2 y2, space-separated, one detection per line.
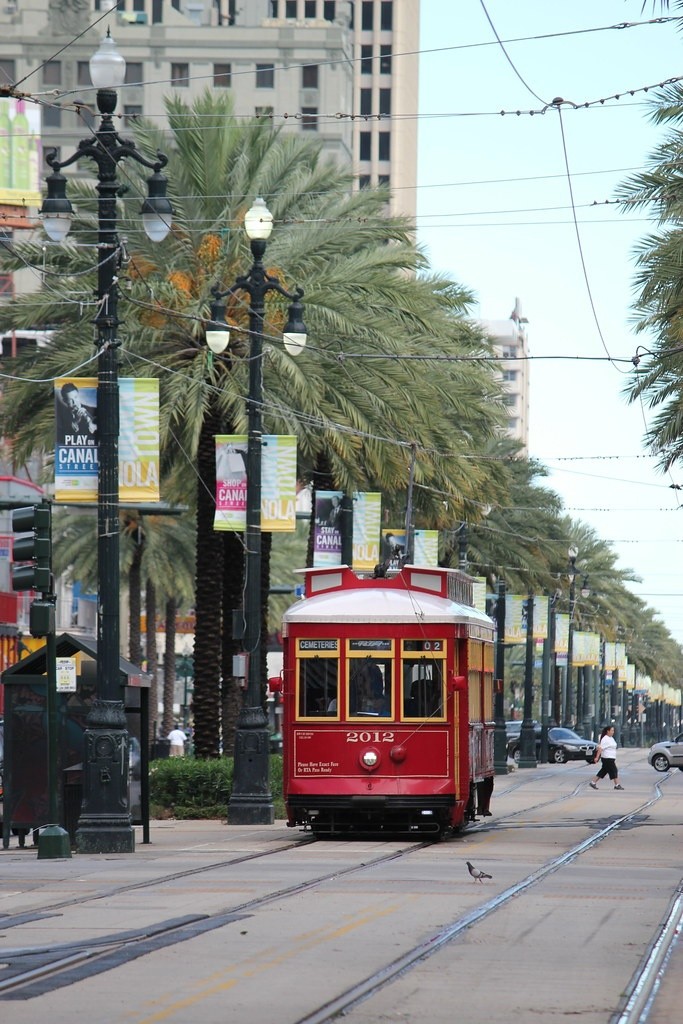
166 725 187 758
57 383 98 443
315 496 342 530
383 533 405 560
590 725 625 791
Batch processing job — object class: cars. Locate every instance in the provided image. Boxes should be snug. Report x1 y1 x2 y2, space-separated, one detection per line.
504 720 542 742
508 728 601 764
648 732 683 772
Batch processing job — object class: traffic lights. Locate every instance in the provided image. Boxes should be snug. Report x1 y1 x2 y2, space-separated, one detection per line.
12 506 36 591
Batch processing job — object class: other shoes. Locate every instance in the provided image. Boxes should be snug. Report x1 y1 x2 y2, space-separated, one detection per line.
614 785 624 790
589 782 598 789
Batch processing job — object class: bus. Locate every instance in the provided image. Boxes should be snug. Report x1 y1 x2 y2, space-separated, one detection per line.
269 564 497 838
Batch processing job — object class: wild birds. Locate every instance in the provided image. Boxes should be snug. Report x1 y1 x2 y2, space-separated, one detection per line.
466 862 492 885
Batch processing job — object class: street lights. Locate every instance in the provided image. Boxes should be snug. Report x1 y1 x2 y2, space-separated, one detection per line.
557 546 591 738
37 21 176 855
458 503 491 571
202 196 308 825
